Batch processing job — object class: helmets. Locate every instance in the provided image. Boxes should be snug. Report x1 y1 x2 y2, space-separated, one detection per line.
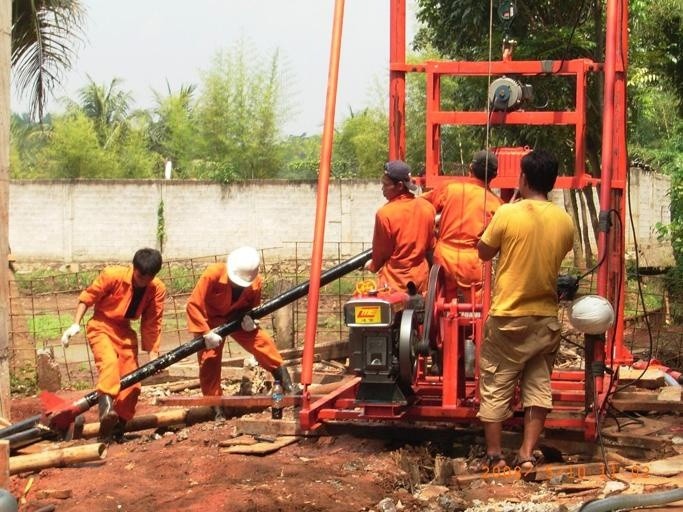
227 246 261 288
567 294 615 336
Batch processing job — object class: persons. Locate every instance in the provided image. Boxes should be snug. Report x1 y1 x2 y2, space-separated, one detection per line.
61 247 166 444
477 149 584 473
361 160 436 297
186 247 303 422
418 150 504 381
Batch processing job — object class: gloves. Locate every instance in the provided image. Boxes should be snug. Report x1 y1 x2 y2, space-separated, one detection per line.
241 314 260 333
358 259 373 271
202 330 224 351
60 323 80 347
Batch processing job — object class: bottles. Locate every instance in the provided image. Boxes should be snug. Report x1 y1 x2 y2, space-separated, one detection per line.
271 380 284 419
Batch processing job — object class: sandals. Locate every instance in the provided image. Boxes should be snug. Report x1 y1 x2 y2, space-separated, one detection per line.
476 448 509 472
511 454 537 481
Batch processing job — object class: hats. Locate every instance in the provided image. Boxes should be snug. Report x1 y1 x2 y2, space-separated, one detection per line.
472 150 498 171
383 159 418 191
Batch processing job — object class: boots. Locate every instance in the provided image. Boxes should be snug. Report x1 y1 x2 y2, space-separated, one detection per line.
429 348 442 374
97 394 130 444
463 339 475 379
271 365 303 395
